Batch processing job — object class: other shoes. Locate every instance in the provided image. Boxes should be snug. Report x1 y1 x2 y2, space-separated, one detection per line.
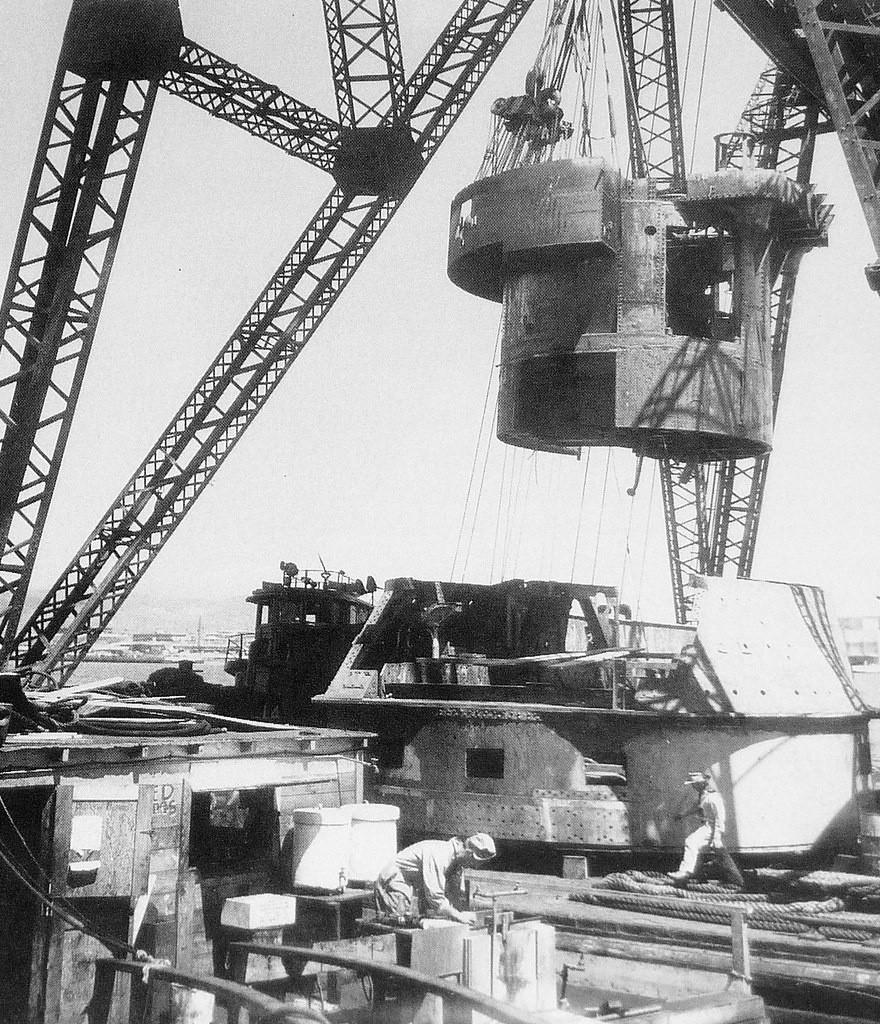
668 871 692 882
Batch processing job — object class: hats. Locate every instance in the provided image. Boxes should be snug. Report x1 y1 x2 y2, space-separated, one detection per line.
685 772 711 788
467 832 497 861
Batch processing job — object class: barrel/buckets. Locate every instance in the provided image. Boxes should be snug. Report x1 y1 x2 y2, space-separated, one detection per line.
293 807 351 891
341 804 400 882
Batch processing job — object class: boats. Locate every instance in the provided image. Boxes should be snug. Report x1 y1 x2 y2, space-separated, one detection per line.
0 0 880 1024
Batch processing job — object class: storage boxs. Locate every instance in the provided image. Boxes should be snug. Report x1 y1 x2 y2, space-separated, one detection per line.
220 892 296 932
355 920 470 981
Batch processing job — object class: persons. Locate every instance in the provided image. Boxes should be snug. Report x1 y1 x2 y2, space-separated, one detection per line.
374 834 497 924
668 772 745 888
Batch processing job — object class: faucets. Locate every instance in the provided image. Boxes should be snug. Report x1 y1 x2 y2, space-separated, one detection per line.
513 881 522 890
472 881 482 897
717 968 753 1022
560 949 586 1001
338 867 347 880
